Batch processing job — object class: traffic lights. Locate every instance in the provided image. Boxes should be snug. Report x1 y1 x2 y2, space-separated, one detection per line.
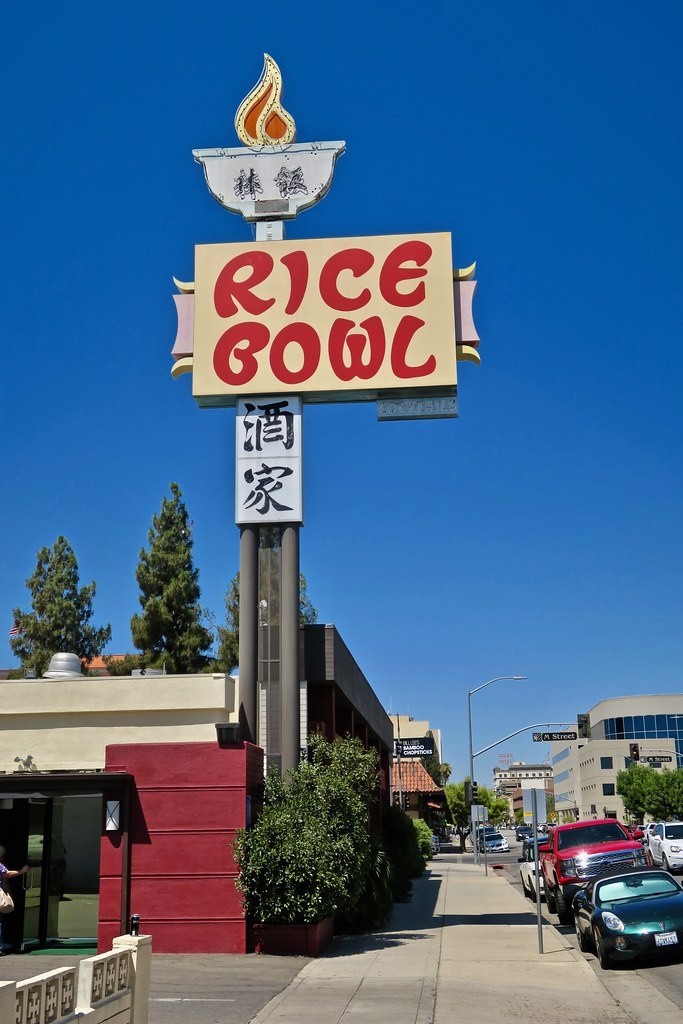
629 742 641 762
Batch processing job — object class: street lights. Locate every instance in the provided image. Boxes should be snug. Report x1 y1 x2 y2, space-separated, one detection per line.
468 676 527 864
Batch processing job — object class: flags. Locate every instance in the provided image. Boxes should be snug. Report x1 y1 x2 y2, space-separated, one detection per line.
9 611 35 636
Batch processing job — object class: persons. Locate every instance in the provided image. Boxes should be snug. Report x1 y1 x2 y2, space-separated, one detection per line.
0 847 32 955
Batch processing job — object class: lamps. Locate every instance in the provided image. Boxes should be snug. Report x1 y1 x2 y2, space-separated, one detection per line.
105 801 120 831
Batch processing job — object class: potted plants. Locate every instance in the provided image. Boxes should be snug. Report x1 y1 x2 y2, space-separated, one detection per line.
229 799 358 955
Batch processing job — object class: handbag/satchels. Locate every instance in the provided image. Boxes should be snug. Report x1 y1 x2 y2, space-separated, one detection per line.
0 887 14 913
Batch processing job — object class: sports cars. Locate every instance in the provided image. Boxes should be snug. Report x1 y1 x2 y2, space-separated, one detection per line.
571 865 683 968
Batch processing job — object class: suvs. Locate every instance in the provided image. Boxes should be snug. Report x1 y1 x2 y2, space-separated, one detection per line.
538 817 654 927
518 836 546 903
648 821 683 872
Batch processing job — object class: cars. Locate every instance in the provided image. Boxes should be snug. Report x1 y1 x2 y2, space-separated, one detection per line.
476 831 511 853
515 826 533 841
478 823 517 830
537 823 558 834
470 825 500 848
621 822 656 845
